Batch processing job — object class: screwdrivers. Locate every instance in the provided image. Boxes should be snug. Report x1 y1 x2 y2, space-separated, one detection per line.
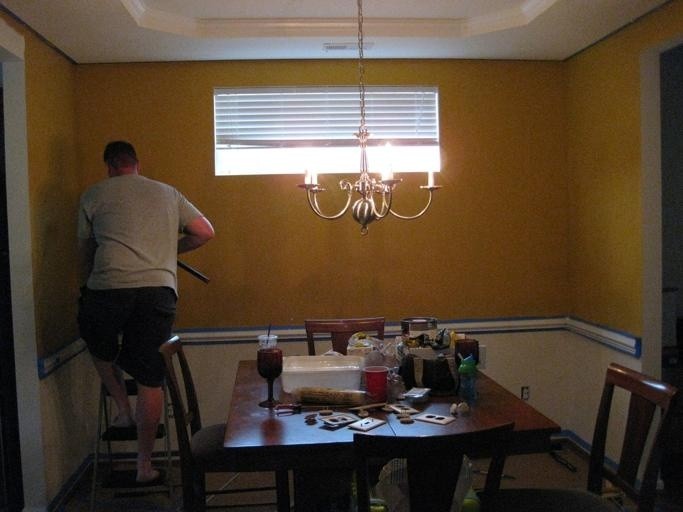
277 404 347 413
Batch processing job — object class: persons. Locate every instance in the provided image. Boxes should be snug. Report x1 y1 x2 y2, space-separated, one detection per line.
69 141 217 485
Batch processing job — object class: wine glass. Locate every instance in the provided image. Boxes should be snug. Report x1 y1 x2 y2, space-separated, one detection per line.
256 349 282 408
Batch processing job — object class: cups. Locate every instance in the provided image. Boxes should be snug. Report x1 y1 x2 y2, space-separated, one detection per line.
365 366 388 402
257 334 278 349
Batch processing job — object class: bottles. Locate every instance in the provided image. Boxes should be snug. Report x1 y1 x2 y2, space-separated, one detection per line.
459 364 476 401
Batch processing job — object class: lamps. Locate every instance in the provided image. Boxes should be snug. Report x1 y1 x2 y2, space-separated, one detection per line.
294 1 443 235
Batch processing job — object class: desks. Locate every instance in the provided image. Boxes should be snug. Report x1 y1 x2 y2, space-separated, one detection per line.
224 357 561 512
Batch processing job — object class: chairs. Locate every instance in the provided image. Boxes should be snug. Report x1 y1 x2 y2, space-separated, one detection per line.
304 317 386 357
158 335 295 512
477 363 683 512
353 422 515 512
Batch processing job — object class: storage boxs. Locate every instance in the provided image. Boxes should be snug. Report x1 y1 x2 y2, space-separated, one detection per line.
281 355 365 393
